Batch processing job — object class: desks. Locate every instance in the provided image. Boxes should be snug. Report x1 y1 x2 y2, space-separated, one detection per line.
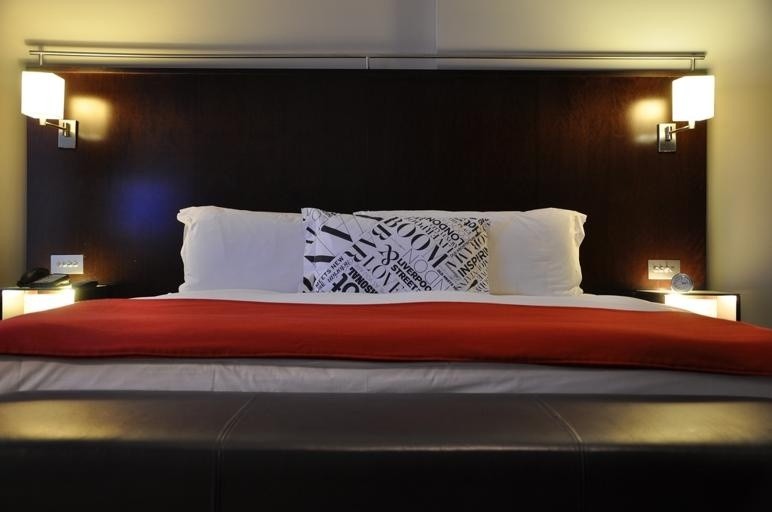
638 291 741 322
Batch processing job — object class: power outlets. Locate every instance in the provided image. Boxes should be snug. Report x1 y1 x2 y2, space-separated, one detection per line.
648 260 681 280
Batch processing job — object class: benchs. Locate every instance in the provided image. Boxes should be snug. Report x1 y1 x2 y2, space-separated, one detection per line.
0 394 772 512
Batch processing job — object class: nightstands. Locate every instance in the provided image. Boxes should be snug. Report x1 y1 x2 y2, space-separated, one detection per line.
1 287 106 320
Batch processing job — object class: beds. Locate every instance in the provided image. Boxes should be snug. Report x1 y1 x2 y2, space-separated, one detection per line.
0 69 771 393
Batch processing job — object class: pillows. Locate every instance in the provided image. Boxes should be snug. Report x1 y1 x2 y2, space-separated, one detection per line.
355 208 585 297
299 215 491 294
176 204 303 290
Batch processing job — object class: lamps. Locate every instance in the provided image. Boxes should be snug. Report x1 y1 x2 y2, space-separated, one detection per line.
20 69 76 150
657 75 716 153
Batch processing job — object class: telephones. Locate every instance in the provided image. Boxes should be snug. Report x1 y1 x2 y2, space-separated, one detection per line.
20 268 70 287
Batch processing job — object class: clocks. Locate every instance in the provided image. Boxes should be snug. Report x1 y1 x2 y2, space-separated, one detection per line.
671 273 694 293
